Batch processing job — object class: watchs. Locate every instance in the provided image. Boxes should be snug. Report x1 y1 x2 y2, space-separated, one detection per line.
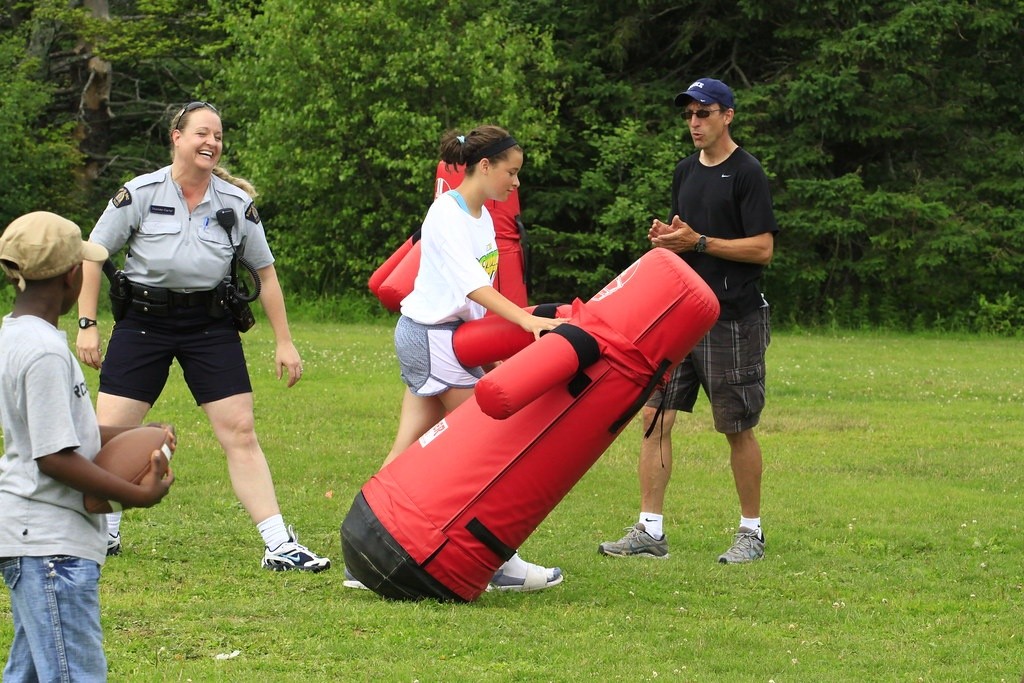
694 234 706 253
77 316 97 329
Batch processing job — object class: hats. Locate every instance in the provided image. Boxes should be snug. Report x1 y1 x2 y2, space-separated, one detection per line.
673 77 738 112
1 207 110 284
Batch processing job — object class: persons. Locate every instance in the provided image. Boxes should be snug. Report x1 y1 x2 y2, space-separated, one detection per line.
75 101 333 577
598 78 780 565
343 124 573 592
0 211 176 683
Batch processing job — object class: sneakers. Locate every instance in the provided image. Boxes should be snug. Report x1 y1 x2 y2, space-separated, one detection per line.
262 525 334 573
716 526 766 567
340 563 371 588
104 531 123 558
484 555 567 594
598 523 672 562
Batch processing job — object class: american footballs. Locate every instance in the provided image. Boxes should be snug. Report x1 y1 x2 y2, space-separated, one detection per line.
83 427 175 516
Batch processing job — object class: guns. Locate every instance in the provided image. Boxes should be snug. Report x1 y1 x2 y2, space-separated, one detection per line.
102 259 129 300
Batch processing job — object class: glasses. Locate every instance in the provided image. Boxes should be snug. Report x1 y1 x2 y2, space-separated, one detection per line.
175 100 221 132
679 108 728 120
